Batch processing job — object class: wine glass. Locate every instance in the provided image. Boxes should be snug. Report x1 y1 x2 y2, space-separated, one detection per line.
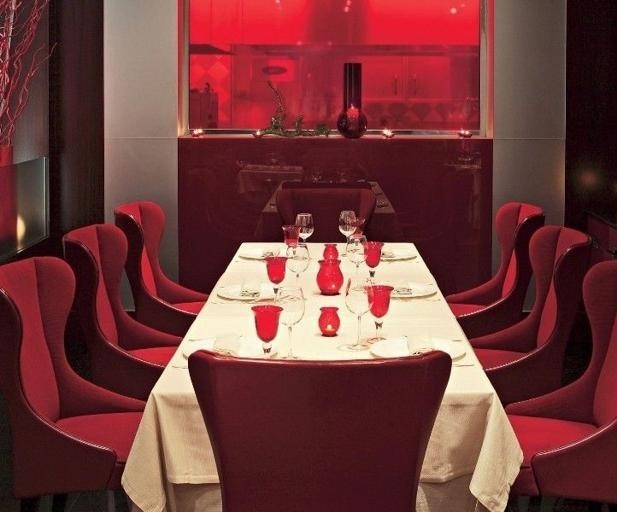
338 210 366 257
273 287 306 361
251 304 283 360
346 235 368 282
281 224 300 251
345 276 375 351
366 285 394 345
286 243 310 299
264 256 288 298
295 213 314 244
364 240 385 285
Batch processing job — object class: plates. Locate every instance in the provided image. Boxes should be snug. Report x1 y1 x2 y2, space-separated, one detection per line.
379 244 417 261
374 197 389 209
370 334 468 361
371 186 383 195
377 279 438 298
182 334 266 361
217 280 279 301
270 201 276 209
239 246 285 260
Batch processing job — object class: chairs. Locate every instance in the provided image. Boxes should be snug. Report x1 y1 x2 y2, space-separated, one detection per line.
187 349 451 510
275 188 376 242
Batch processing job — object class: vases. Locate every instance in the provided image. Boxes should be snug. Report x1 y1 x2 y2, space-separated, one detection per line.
337 62 367 139
